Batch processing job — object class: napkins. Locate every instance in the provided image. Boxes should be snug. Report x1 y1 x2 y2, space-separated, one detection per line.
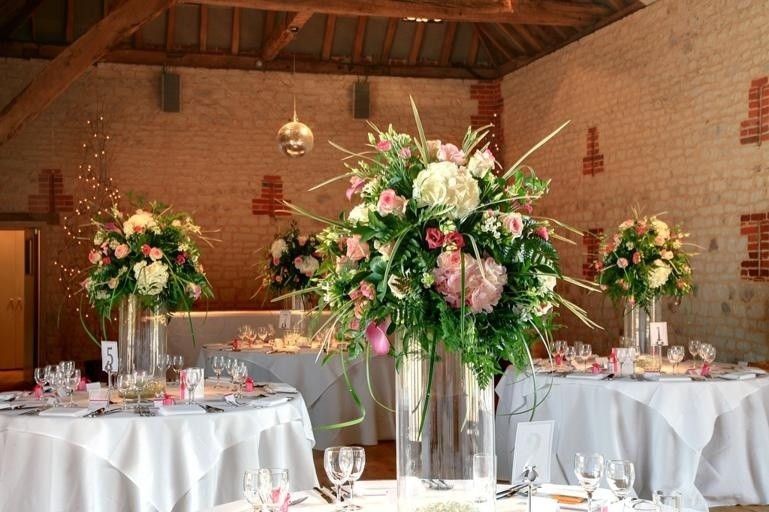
39 407 88 417
268 382 296 393
735 366 766 374
537 482 612 500
644 374 691 383
158 405 206 416
719 372 756 381
2 403 23 410
249 396 288 407
566 371 609 381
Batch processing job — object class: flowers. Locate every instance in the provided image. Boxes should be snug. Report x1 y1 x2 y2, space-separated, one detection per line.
65 180 214 350
589 201 699 310
261 99 583 418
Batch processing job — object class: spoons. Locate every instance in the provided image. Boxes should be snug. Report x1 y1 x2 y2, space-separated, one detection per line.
525 470 537 512
496 482 527 499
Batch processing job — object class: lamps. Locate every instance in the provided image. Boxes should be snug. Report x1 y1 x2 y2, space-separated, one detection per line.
275 27 315 161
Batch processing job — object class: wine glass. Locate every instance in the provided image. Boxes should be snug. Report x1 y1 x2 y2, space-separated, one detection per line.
116 371 146 414
668 341 716 378
35 361 82 410
237 326 293 350
549 340 591 375
244 468 288 512
614 345 641 379
573 454 634 512
172 353 185 385
211 356 249 403
184 366 202 413
324 446 366 511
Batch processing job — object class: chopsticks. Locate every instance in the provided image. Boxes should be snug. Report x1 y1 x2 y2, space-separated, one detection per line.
90 408 122 417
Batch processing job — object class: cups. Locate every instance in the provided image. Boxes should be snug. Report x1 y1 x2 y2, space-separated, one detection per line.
472 453 497 503
653 488 682 512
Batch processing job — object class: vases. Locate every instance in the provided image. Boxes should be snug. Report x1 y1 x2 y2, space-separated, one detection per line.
381 319 507 512
111 292 167 394
623 290 667 373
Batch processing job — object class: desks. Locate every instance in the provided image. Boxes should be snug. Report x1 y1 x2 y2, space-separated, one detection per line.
0 378 302 512
506 357 768 505
168 310 339 371
209 479 690 511
196 334 398 450
